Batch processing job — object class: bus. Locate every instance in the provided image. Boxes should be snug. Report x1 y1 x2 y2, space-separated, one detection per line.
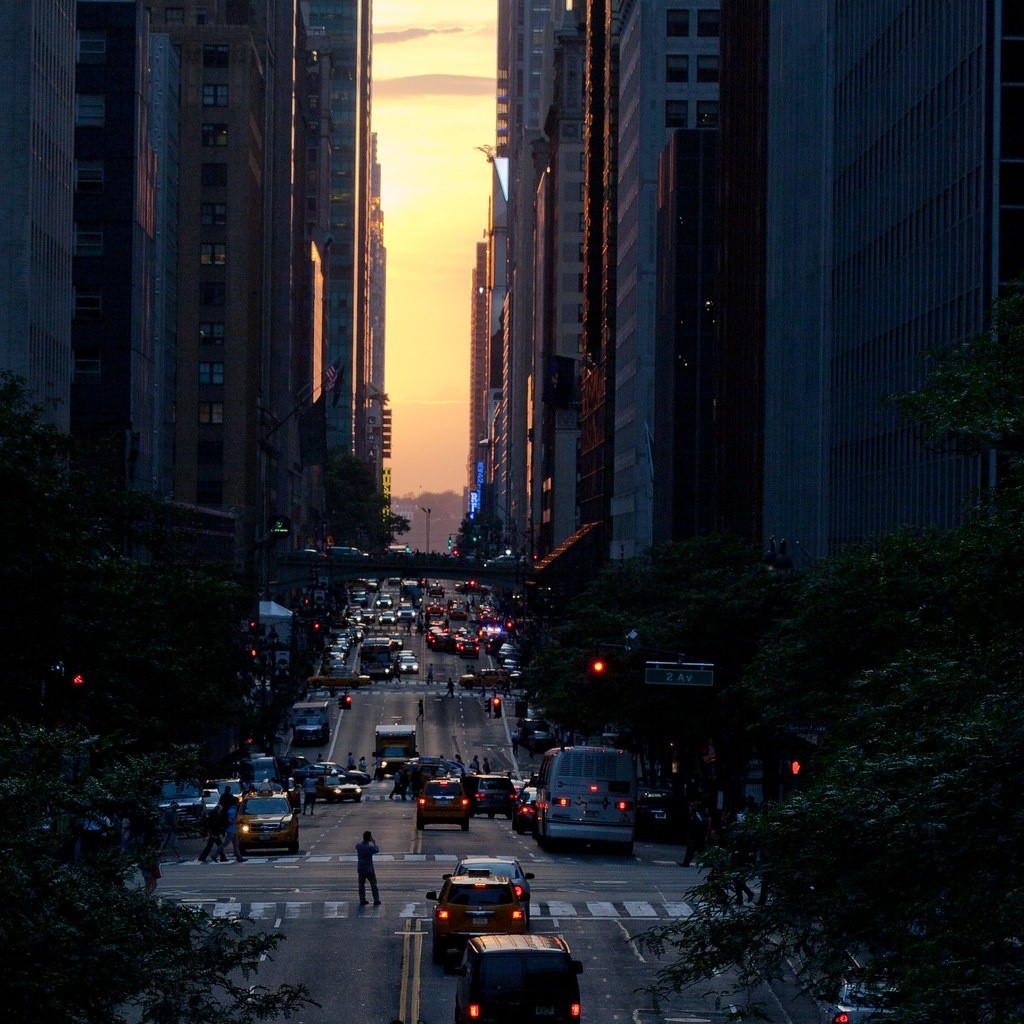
358 638 394 679
530 744 636 853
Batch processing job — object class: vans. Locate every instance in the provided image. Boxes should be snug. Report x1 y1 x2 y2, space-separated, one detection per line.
241 758 279 791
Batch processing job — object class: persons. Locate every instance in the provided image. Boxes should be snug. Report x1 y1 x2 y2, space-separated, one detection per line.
159 784 256 864
427 663 434 684
466 663 511 698
511 729 520 754
367 548 495 561
388 766 421 800
141 846 162 896
347 752 354 770
359 753 383 781
439 754 491 775
355 830 382 906
678 794 756 905
415 699 426 721
316 753 324 762
362 579 492 634
302 773 319 815
446 677 455 697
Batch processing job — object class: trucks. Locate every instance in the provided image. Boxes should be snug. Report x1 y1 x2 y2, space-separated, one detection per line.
290 702 331 744
372 725 417 777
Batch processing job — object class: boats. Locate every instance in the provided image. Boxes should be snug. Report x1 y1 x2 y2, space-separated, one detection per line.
405 548 409 552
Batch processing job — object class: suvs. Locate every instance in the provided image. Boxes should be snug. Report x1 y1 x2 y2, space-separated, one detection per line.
426 875 527 958
416 778 473 832
464 774 516 819
454 934 582 1024
234 778 300 852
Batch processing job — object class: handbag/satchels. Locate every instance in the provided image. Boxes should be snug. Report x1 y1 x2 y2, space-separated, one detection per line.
151 865 161 880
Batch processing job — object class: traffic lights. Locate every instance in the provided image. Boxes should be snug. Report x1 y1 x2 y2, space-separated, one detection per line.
590 658 607 693
338 696 351 710
493 698 502 718
448 539 451 550
484 698 491 718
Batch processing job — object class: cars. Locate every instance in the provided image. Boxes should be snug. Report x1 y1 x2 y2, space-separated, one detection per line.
302 545 528 689
511 778 538 834
160 777 241 832
820 981 900 1024
313 768 362 802
401 755 478 795
278 756 372 786
442 859 534 919
516 717 556 750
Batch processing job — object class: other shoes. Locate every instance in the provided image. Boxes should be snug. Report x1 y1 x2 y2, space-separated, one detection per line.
360 901 369 906
676 861 690 867
747 891 755 903
198 857 207 863
374 901 381 906
732 901 744 906
211 855 218 863
220 858 229 862
238 858 248 862
